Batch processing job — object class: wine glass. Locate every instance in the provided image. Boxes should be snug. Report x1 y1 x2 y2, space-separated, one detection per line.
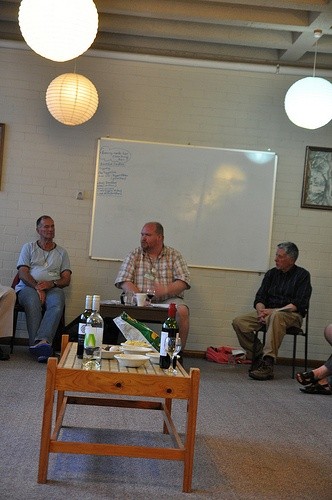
164 337 182 376
147 288 155 306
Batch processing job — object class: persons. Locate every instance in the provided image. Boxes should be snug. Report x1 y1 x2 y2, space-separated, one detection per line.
114 221 192 369
232 242 313 381
295 324 332 395
0 216 73 364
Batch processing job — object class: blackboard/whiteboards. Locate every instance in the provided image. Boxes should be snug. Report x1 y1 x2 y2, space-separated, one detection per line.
89 138 277 273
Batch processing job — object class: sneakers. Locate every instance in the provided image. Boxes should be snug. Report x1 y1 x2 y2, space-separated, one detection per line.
249 359 273 379
249 358 260 371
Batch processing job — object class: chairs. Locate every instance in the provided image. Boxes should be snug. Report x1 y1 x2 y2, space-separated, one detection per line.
11 271 65 353
252 303 309 379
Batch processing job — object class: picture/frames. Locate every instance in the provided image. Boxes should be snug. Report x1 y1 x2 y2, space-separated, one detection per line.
300 145 332 210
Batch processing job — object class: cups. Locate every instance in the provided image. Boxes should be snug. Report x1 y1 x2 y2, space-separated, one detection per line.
125 291 134 305
135 293 146 307
83 346 101 371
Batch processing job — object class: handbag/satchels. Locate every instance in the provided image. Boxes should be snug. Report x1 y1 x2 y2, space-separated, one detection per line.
205 347 252 364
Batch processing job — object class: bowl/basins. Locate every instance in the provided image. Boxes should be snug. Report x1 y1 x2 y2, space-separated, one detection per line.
146 353 180 366
101 346 124 359
121 342 153 355
114 353 150 367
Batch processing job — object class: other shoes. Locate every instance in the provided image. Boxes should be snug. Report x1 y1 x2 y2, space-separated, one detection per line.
35 343 51 362
29 346 35 352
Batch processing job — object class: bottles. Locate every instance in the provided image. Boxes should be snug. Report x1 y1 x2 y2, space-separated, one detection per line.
76 295 93 359
159 304 179 369
82 295 104 364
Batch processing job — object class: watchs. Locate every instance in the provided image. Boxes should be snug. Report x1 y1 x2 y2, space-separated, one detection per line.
52 280 57 288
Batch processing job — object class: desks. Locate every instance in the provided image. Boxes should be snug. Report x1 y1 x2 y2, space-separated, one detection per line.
100 302 169 323
37 335 201 493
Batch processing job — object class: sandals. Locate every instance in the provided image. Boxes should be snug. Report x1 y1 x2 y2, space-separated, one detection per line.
296 370 319 384
298 380 331 393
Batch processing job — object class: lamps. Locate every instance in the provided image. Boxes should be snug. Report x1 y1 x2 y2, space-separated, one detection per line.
18 0 99 63
283 29 332 129
45 60 98 126
